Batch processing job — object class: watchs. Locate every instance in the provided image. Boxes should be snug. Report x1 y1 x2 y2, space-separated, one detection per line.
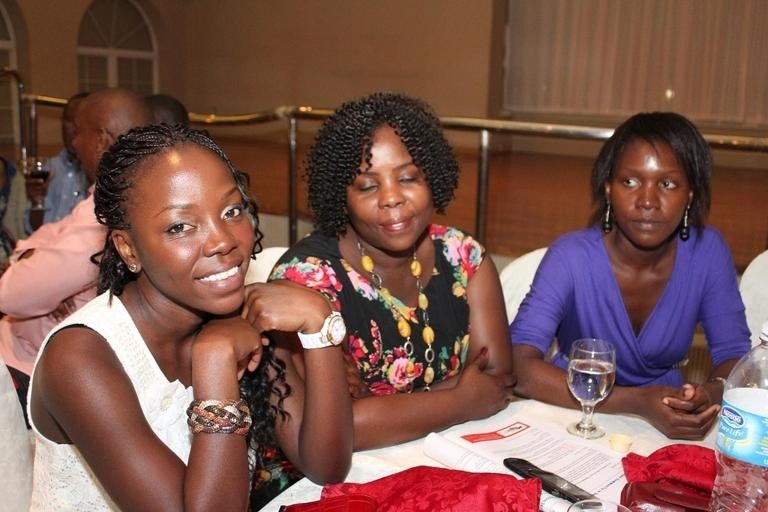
297 310 347 349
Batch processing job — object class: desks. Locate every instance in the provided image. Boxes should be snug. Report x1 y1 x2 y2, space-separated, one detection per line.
258 398 720 511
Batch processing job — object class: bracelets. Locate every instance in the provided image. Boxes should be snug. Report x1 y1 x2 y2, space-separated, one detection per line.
185 398 253 437
710 376 726 385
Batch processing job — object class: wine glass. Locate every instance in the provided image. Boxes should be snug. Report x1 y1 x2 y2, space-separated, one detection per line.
19 156 51 215
567 338 616 439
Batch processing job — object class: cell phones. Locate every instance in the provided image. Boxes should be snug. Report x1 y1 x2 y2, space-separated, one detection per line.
504 456 602 510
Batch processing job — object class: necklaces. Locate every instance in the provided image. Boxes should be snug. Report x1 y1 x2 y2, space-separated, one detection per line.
357 241 435 394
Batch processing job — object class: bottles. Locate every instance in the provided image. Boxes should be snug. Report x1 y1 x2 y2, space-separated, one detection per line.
709 323 768 511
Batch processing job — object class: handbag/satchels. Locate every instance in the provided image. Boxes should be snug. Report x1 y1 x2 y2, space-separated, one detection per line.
619 477 712 511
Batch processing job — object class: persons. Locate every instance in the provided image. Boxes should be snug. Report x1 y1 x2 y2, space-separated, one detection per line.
509 112 752 440
267 93 518 451
26 123 353 512
1 86 187 428
